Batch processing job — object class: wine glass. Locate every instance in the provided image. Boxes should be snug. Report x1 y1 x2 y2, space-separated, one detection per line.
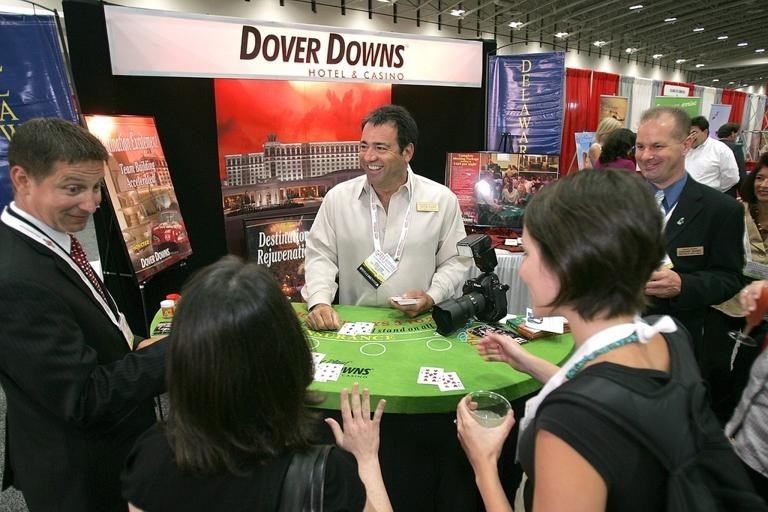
728 279 768 348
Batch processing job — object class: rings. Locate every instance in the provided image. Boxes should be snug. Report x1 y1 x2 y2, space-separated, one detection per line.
740 288 747 292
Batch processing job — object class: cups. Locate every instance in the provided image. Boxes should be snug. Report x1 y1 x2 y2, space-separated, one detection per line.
464 391 513 427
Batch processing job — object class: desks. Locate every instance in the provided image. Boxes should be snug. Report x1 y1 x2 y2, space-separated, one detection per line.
469 233 536 315
149 300 578 416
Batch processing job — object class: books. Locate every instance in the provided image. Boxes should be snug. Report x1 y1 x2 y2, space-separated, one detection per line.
506 310 572 341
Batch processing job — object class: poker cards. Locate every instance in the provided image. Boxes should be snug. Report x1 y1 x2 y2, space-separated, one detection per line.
417 367 444 385
354 322 374 335
311 352 344 382
438 372 465 391
391 295 422 305
338 322 356 336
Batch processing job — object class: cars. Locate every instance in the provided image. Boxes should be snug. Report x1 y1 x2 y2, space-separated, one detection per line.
151 218 189 251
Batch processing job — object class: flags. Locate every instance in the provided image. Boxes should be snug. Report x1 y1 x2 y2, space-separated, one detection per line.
707 104 732 139
0 12 90 221
654 96 699 118
597 95 627 128
485 50 567 158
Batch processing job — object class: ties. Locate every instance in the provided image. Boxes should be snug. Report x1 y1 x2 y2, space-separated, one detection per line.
654 190 666 219
66 233 109 303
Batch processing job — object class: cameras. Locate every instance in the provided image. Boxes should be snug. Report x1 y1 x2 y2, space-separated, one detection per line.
431 234 509 335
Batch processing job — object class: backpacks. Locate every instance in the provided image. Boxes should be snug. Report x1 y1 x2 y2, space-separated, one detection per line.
538 316 767 512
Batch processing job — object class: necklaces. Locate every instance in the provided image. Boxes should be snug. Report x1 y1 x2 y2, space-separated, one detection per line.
751 203 767 235
566 322 649 380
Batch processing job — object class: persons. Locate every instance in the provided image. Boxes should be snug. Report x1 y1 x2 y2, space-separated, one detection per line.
586 115 747 199
456 165 767 511
119 254 396 510
301 103 476 333
0 115 175 510
734 151 767 266
722 275 768 476
479 163 556 221
634 105 747 334
